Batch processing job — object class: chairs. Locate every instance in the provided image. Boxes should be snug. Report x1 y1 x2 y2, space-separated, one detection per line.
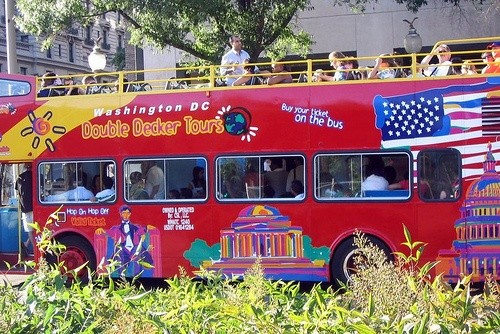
47 67 457 96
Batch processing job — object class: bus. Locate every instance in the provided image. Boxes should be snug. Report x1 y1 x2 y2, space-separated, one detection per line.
1 51 500 294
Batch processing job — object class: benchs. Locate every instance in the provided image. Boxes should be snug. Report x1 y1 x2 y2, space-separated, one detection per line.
359 190 408 198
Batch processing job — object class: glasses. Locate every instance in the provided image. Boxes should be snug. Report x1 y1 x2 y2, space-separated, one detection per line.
439 51 446 52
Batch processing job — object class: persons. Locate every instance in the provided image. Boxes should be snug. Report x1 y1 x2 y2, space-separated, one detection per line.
416 148 462 202
220 34 294 87
487 41 500 62
315 51 367 81
14 162 34 258
451 57 464 75
125 159 208 201
317 154 411 200
421 44 456 77
37 72 129 97
461 60 479 75
367 53 397 79
217 156 306 201
481 52 500 74
39 163 118 203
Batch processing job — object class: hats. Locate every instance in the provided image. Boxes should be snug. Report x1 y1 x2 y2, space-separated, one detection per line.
482 53 492 58
489 41 500 48
